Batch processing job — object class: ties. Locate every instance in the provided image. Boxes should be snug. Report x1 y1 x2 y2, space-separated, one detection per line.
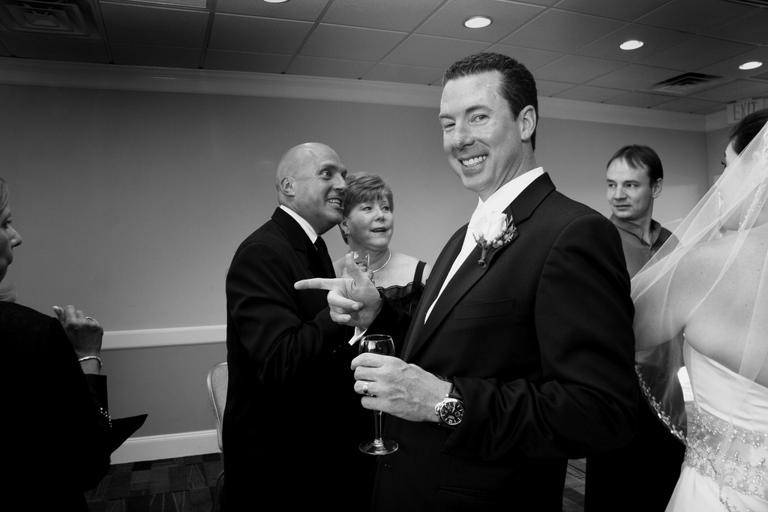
314 235 336 278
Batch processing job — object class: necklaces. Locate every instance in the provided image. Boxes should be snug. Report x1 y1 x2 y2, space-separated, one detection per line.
368 250 393 272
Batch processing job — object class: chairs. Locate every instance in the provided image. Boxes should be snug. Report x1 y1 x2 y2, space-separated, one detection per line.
79 354 104 378
206 359 228 511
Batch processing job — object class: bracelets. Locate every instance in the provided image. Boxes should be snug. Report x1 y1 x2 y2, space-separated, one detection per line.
79 355 103 368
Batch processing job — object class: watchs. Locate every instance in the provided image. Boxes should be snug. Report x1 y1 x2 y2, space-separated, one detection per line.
436 382 466 430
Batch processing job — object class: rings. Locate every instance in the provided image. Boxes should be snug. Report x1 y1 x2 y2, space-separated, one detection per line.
362 378 371 395
85 316 94 320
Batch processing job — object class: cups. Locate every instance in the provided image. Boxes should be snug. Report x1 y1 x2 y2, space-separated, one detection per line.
346 247 370 277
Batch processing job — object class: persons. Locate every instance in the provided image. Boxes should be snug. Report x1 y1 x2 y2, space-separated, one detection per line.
585 145 690 511
331 171 433 361
222 141 377 510
293 52 634 509
628 107 767 510
0 179 149 511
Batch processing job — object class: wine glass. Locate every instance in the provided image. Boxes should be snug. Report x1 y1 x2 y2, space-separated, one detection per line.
357 333 399 457
